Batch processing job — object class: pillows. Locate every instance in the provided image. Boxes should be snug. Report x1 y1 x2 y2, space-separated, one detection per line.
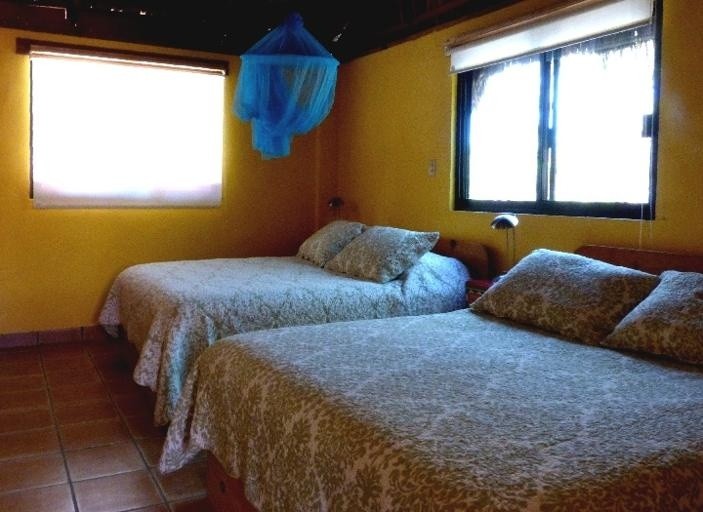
601 269 703 368
297 217 440 285
470 247 660 345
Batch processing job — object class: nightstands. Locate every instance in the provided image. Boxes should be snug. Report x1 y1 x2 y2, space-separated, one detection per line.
466 276 497 308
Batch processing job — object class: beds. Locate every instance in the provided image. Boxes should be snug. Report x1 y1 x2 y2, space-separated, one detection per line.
151 243 703 510
98 239 500 437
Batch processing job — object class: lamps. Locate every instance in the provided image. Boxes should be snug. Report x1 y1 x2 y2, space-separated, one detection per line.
327 197 345 218
489 214 519 275
